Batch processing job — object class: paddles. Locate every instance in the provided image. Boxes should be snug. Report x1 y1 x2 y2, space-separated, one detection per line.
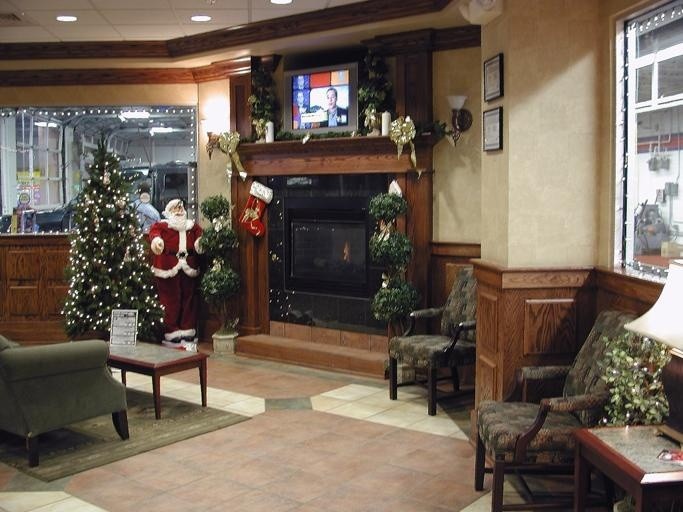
0 386 254 482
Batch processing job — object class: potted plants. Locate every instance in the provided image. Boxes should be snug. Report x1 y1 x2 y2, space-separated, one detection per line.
482 53 504 152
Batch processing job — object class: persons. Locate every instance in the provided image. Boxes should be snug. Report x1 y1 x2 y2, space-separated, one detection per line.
318 88 348 130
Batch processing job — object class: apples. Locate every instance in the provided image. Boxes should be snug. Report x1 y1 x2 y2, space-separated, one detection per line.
381 110 391 137
265 120 274 143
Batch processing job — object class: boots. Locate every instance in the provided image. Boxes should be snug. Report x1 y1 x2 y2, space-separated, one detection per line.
0 160 197 234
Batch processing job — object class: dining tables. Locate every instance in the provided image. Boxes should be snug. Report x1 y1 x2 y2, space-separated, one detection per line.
370 193 421 384
198 193 239 355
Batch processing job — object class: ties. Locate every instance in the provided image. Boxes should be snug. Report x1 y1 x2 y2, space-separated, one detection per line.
283 61 358 135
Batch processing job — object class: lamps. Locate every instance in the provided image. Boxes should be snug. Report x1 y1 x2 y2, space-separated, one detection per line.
0 334 130 468
388 267 477 418
475 308 632 512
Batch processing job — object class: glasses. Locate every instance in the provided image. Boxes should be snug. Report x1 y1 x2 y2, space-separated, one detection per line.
446 94 473 147
621 260 683 445
